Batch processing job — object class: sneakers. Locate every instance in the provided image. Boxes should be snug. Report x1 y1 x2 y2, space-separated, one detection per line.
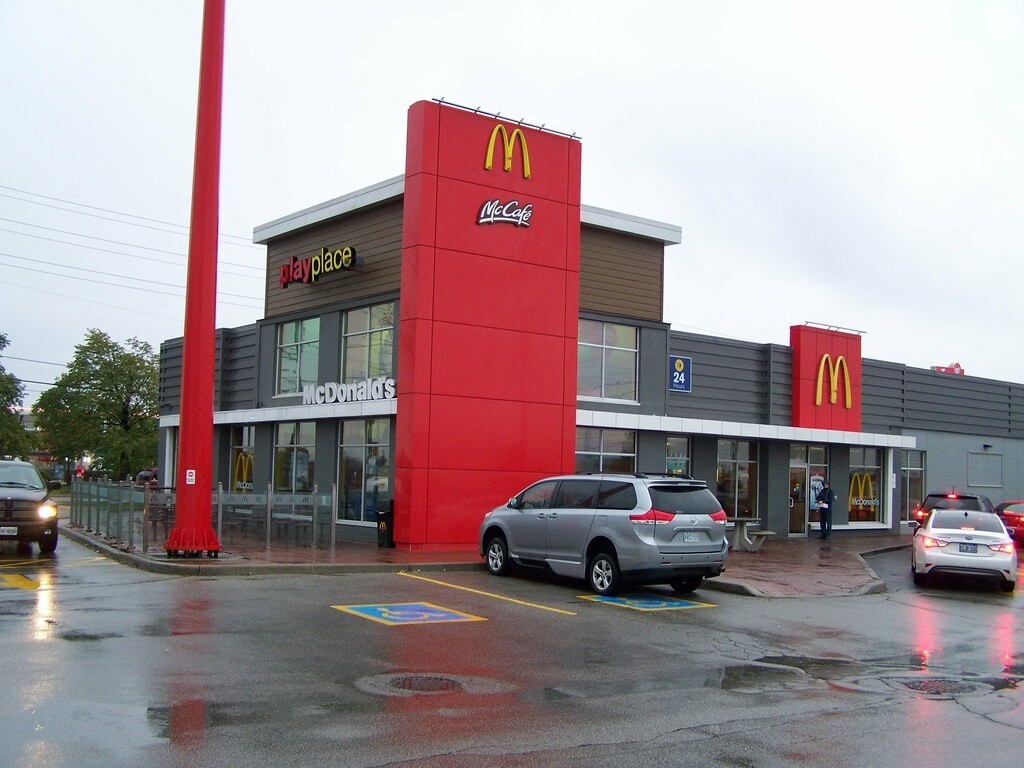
817 535 824 539
825 534 832 540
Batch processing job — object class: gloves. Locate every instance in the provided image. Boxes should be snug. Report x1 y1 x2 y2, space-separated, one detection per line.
818 501 823 504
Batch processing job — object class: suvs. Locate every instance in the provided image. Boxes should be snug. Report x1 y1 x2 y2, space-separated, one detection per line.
476 471 730 593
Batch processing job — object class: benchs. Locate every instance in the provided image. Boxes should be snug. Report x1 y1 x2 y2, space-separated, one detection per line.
725 529 777 552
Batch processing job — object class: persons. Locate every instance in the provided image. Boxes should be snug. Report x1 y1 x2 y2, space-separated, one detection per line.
816 481 835 540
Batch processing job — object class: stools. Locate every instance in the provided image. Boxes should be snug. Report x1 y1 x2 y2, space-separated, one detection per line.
149 504 329 547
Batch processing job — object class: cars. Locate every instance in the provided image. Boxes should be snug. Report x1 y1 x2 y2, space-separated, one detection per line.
994 499 1024 537
136 467 157 489
0 460 63 552
912 492 1004 534
717 477 750 511
906 510 1018 592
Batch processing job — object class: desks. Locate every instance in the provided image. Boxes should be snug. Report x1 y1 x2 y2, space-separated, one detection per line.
727 518 762 551
